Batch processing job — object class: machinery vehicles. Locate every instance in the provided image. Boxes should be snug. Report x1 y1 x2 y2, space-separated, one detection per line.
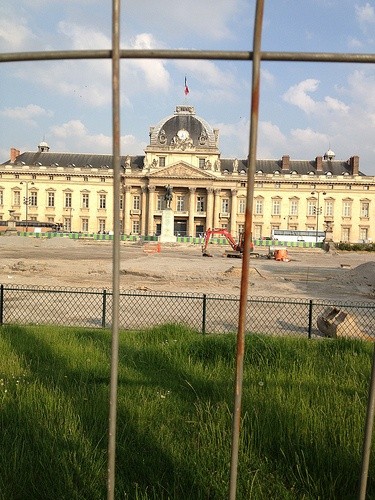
200 226 258 258
267 243 291 261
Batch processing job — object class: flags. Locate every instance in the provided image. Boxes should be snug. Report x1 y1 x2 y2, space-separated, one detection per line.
184 77 189 95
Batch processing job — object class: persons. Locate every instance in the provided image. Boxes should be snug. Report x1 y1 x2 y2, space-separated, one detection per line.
165 183 174 208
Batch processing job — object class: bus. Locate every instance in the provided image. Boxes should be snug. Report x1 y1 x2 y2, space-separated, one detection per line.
271 229 325 242
